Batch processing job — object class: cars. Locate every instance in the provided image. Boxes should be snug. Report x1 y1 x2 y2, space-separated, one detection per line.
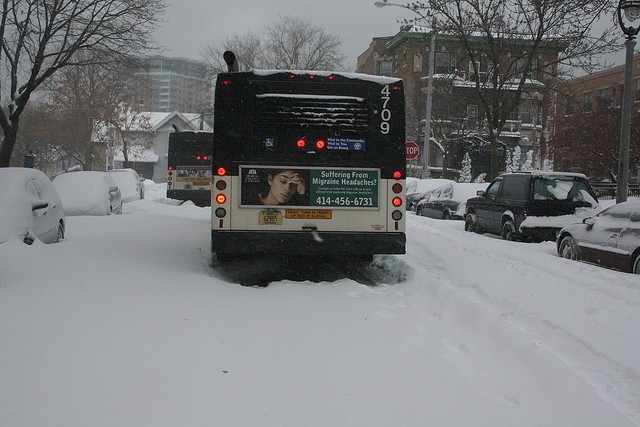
417 183 488 220
406 179 456 212
109 168 146 201
52 171 123 214
556 199 640 274
0 168 65 245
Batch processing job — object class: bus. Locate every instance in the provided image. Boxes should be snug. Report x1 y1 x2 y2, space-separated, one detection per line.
211 50 406 270
167 124 214 206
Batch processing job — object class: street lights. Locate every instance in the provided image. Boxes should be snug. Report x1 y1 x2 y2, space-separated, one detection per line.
374 2 437 178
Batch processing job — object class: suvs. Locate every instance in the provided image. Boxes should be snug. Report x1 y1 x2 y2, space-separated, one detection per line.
465 172 601 244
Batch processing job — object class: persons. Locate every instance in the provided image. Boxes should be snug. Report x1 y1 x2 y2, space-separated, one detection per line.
242 169 309 207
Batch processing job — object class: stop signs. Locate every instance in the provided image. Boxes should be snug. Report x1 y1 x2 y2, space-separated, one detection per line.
405 141 420 160
